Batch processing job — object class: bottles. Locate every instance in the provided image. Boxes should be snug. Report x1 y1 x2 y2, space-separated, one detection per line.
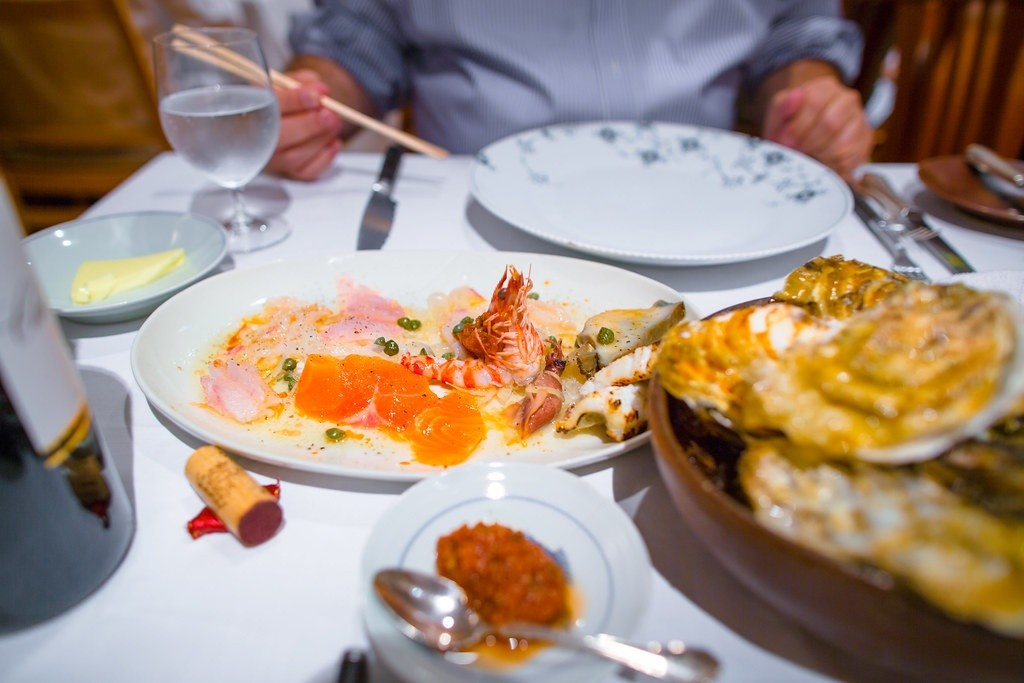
3 170 135 634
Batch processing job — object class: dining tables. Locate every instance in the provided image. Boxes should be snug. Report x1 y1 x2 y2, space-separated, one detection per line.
0 151 1024 683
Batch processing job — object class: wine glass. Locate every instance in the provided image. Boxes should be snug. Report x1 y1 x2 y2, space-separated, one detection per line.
151 28 291 256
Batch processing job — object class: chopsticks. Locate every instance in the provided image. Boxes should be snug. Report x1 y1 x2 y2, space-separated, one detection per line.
171 25 448 163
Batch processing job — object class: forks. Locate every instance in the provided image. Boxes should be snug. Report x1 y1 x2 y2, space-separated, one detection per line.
844 181 932 285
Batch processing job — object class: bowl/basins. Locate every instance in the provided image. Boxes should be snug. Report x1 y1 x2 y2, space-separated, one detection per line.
653 297 1023 682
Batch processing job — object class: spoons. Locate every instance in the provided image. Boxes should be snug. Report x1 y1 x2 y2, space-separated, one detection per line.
367 561 719 683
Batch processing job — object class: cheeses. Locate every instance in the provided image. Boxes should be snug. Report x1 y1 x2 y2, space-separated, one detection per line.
70 248 186 302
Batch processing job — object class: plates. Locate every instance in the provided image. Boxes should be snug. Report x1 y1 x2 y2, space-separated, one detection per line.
468 118 852 271
356 460 656 683
919 155 1022 225
131 245 702 478
21 210 228 326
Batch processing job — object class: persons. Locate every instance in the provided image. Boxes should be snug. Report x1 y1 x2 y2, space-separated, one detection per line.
266 0 875 183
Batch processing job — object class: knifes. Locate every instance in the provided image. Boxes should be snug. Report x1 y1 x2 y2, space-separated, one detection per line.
357 143 404 249
854 162 976 275
